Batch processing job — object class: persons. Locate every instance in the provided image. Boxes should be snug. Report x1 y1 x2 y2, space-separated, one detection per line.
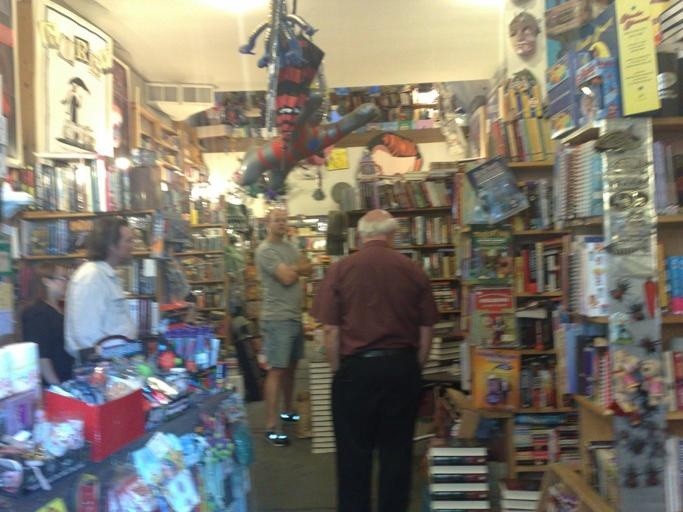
62 217 135 379
509 12 538 55
255 207 309 447
224 233 247 316
312 209 440 512
19 262 73 387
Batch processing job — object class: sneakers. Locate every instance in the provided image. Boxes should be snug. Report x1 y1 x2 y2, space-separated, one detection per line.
264 431 288 447
280 411 300 422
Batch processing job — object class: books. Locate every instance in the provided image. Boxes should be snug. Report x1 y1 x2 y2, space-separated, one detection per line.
400 248 460 311
378 181 461 246
464 155 532 412
422 413 566 512
512 181 564 294
420 319 464 376
515 298 565 408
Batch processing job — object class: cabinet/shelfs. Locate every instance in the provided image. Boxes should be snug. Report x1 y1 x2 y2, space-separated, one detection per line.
245 216 330 362
342 166 458 441
165 222 231 353
131 86 183 175
177 120 208 184
19 208 162 343
462 159 574 481
560 119 683 511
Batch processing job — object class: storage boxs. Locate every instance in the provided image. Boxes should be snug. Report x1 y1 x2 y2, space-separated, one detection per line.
43 374 146 463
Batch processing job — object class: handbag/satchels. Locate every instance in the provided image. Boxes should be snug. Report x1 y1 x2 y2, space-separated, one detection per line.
80 336 144 364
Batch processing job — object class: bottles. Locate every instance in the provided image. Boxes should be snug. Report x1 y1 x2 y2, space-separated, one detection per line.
520 355 555 408
163 326 220 371
